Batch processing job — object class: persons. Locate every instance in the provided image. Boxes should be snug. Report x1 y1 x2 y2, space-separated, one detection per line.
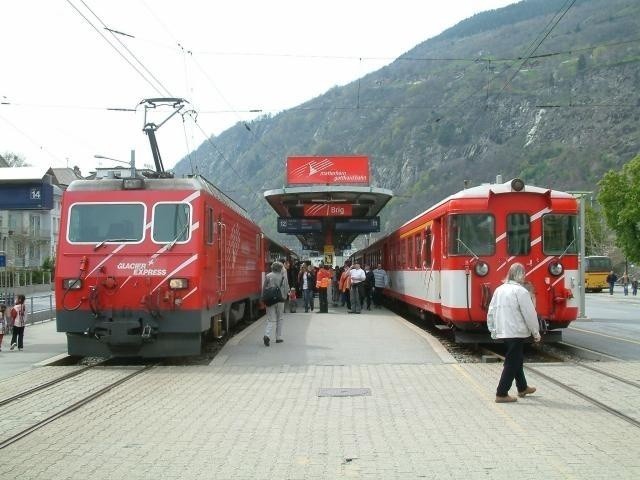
607 270 617 294
9 294 27 351
0 303 9 352
631 278 639 295
279 256 388 312
487 263 541 402
617 272 630 296
261 262 288 346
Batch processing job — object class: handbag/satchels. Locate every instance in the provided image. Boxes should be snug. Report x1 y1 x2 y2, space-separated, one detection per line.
263 287 282 306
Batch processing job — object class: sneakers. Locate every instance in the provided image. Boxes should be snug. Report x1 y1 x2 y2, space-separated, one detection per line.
0 343 23 352
518 386 536 396
291 303 328 313
264 335 283 346
496 395 517 402
332 299 381 313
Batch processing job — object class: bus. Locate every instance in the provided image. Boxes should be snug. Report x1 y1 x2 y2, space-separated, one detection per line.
584 255 612 293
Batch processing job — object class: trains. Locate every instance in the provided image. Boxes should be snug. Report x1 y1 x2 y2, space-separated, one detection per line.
54 177 303 362
340 174 588 351
